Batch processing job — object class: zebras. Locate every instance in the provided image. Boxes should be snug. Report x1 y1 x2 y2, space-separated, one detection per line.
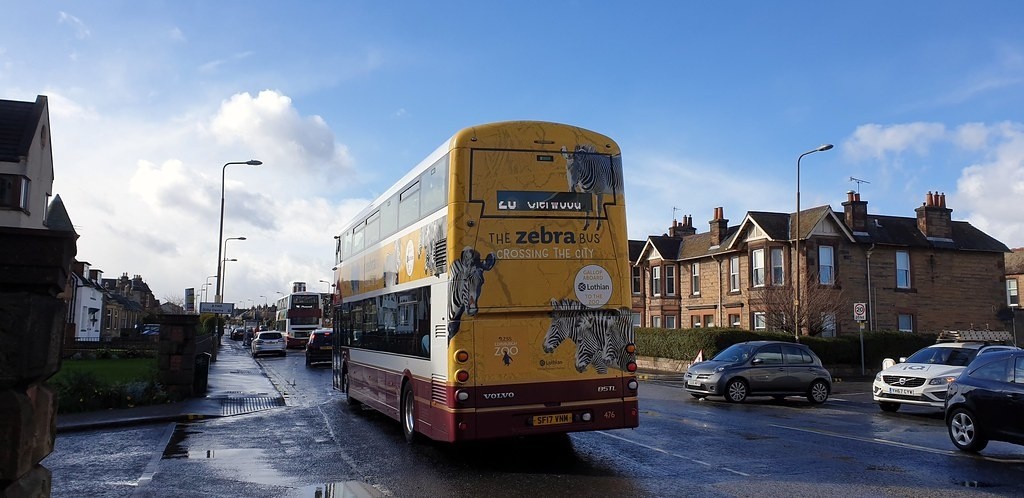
382 238 402 289
560 144 624 232
417 215 447 279
448 245 496 347
542 297 635 375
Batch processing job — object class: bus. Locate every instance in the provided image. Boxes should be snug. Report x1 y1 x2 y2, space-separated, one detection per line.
275 292 324 349
331 120 641 446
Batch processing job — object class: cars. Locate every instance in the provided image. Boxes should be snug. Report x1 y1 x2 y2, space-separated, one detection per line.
251 331 287 359
871 342 1021 412
306 328 333 365
231 328 245 341
943 350 1024 452
683 341 832 405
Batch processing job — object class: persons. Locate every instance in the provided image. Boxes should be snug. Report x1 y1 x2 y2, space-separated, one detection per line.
742 350 751 359
940 350 950 360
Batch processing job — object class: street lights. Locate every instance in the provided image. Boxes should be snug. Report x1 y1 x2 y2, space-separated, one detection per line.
239 291 284 308
320 279 330 295
220 237 247 317
795 144 835 341
208 160 264 362
195 258 237 313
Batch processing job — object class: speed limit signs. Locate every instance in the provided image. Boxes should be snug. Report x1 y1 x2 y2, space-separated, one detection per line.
854 303 866 320
